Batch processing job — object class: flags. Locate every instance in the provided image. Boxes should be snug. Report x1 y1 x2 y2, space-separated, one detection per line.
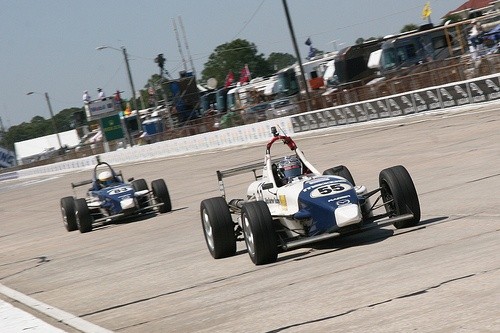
421 2 432 20
239 68 250 85
224 72 234 89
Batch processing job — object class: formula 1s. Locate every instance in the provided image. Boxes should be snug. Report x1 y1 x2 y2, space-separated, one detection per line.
200 124 422 266
61 154 172 233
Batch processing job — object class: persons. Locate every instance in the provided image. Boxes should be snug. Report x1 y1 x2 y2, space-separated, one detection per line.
98 87 107 101
83 89 93 105
276 157 305 185
97 171 115 187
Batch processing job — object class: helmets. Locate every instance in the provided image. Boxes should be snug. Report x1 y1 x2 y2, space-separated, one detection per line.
99 171 114 187
276 155 303 184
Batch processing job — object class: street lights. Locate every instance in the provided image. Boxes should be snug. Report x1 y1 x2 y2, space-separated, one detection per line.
25 91 65 155
96 46 143 135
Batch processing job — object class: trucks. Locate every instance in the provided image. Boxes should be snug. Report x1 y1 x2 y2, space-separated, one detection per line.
199 8 500 124
